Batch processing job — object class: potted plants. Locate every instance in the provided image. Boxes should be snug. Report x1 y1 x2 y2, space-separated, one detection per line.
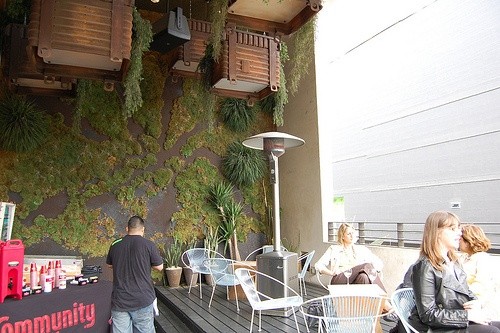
161 243 183 287
204 236 220 284
182 242 199 285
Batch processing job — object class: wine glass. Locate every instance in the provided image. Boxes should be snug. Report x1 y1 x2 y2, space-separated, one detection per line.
367 269 377 289
344 267 352 286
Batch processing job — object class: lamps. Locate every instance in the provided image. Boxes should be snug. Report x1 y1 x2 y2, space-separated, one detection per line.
149 0 192 55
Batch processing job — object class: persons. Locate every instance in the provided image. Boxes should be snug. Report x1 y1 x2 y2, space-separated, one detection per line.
106 216 164 333
390 211 500 333
314 223 396 321
459 225 500 321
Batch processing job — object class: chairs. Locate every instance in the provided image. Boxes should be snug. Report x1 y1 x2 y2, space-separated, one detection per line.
183 248 230 299
392 288 431 333
299 293 394 333
203 258 256 313
298 249 316 295
235 267 310 333
316 265 383 289
245 245 288 261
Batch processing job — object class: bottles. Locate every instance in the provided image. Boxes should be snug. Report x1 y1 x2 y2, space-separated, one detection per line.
30 260 67 292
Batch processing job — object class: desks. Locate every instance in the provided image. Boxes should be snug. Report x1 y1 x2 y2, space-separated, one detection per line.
229 261 256 300
326 283 388 333
